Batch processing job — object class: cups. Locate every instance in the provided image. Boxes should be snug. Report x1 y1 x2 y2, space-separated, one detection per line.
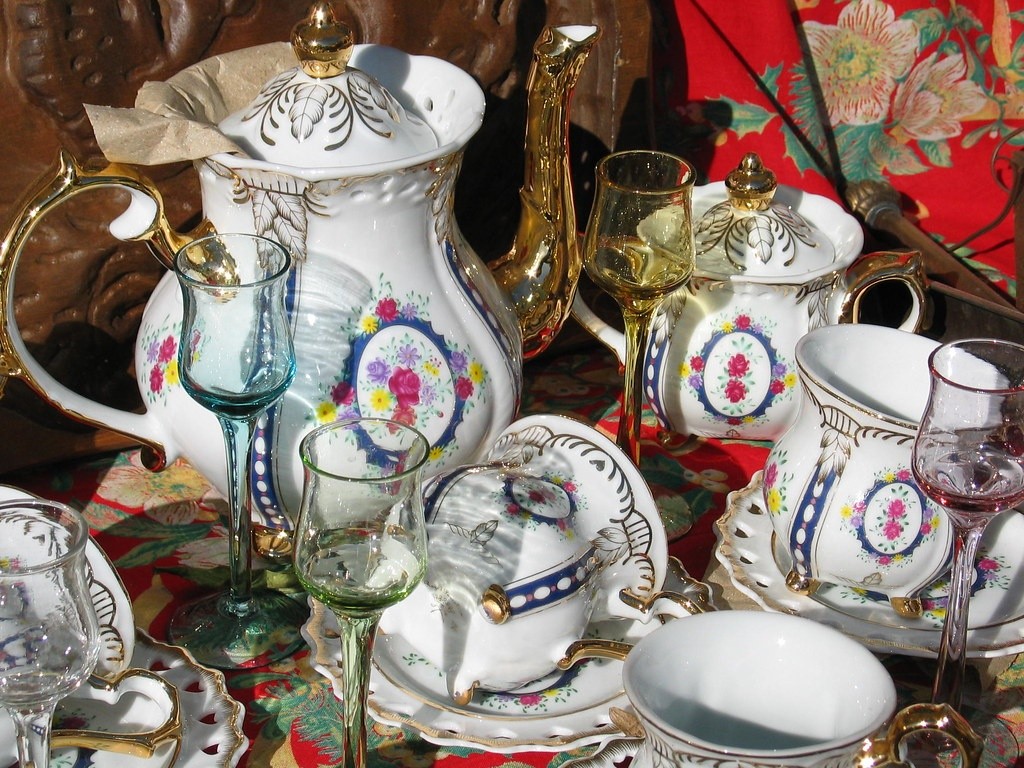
760 321 1010 619
569 153 932 442
375 413 706 707
621 609 986 768
0 484 182 768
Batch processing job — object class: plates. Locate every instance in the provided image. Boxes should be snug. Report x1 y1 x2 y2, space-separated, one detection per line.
557 732 915 768
298 555 719 754
712 469 1024 659
0 629 250 768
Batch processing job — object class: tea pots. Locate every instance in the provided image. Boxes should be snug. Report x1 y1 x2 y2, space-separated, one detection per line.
0 0 602 532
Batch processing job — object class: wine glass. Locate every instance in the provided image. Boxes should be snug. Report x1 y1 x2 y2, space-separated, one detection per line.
0 498 102 768
292 414 430 768
164 230 309 672
911 336 1024 709
580 148 697 543
